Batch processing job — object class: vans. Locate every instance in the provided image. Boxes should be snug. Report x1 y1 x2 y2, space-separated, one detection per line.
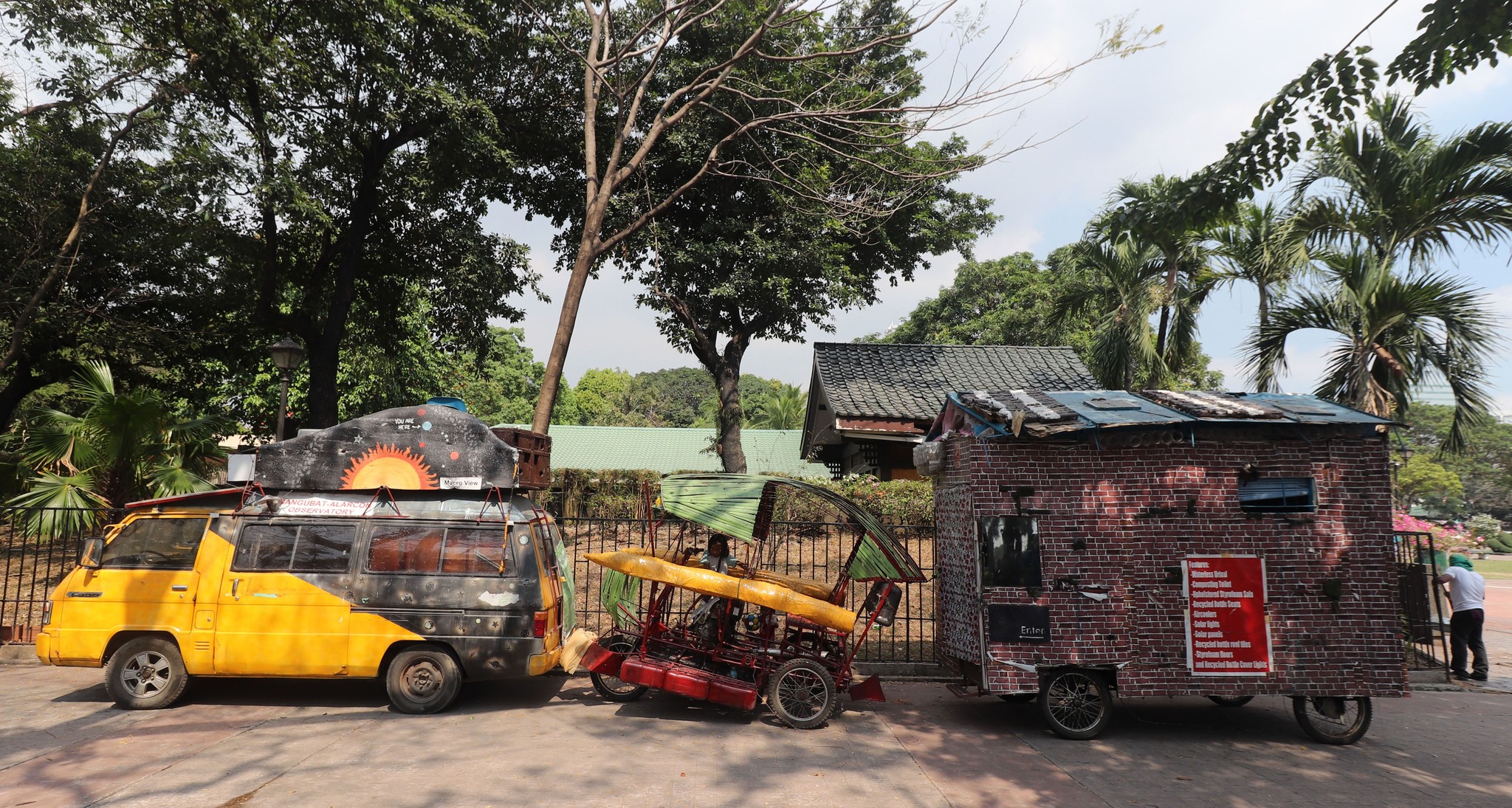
31 402 573 711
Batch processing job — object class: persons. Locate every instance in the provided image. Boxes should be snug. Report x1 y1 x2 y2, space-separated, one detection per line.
1434 554 1489 681
700 534 754 577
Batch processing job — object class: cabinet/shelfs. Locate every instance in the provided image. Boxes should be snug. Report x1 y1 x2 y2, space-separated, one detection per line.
490 427 552 488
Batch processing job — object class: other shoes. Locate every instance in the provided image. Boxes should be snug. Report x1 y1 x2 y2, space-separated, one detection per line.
1450 671 1469 681
1469 671 1488 681
